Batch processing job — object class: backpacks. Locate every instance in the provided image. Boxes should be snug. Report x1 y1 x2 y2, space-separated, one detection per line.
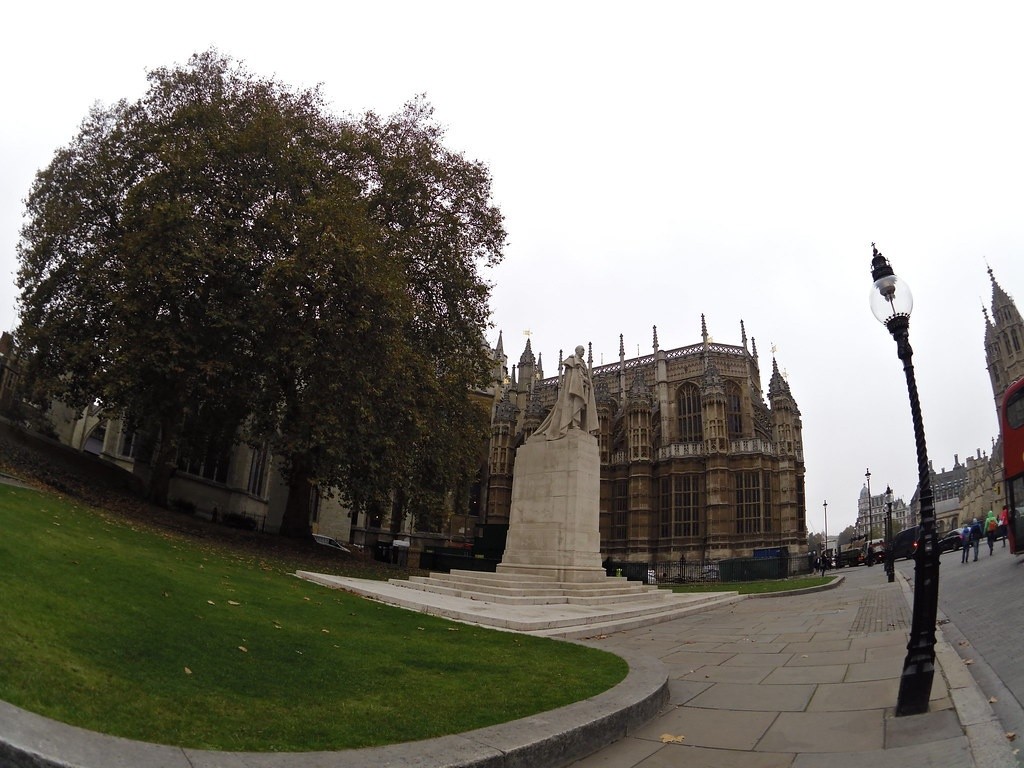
973 523 981 538
990 520 996 531
965 532 970 543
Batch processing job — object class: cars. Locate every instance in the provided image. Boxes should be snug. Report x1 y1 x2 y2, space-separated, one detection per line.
935 529 965 554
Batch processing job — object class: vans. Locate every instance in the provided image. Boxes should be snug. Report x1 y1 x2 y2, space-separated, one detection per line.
893 525 923 561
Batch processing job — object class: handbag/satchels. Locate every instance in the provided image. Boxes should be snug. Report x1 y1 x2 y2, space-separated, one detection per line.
820 564 826 569
998 516 1004 528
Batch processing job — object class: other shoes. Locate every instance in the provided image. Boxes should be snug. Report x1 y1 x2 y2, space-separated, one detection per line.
965 561 968 563
990 554 992 556
973 560 977 562
961 561 964 563
1001 546 1006 548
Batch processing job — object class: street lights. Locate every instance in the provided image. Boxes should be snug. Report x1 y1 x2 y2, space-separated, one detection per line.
823 500 828 557
865 469 873 568
885 486 896 583
866 242 938 717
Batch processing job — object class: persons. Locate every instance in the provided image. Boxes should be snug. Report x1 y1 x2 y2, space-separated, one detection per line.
983 510 999 556
999 505 1009 548
534 345 600 441
868 540 886 566
969 518 984 562
961 524 970 563
